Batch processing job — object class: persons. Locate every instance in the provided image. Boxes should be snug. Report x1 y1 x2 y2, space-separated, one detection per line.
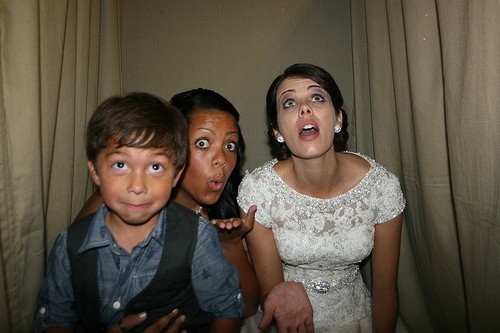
73 88 260 333
33 91 245 333
236 63 406 333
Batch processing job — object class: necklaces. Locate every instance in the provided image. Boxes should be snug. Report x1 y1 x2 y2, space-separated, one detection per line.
196 206 202 214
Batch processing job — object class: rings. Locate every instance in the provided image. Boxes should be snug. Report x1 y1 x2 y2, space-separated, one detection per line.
118 320 126 332
